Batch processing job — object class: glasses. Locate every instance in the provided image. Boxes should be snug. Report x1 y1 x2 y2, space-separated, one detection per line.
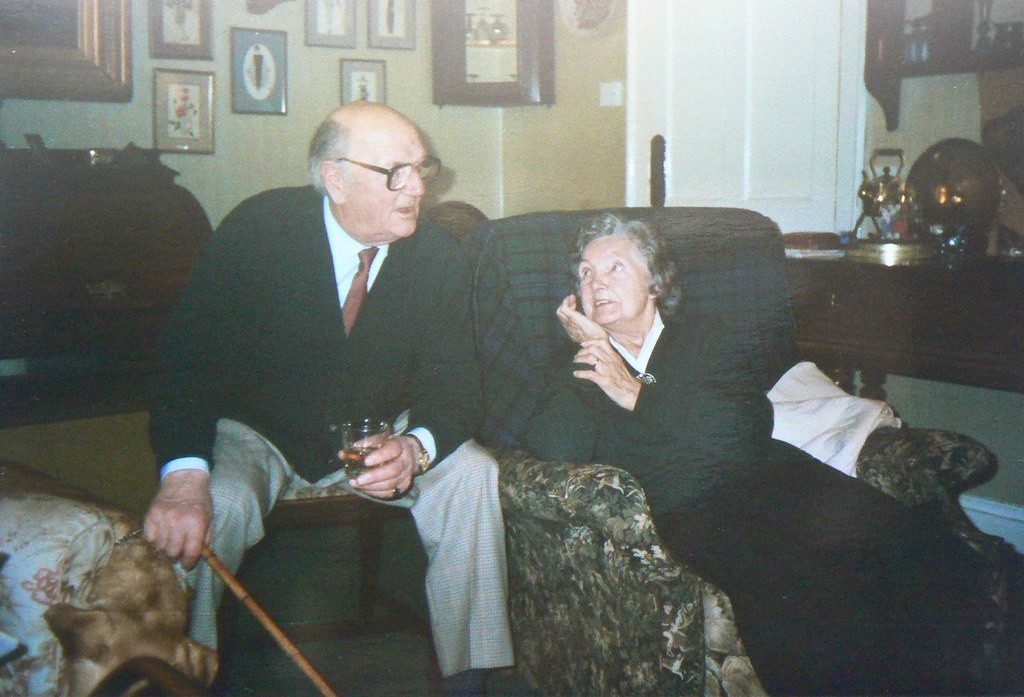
339 157 441 191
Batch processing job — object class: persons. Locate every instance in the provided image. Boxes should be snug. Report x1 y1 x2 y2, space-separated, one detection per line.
141 102 516 697
523 214 1024 697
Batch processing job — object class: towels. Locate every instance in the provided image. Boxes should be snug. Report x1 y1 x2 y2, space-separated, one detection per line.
764 360 903 481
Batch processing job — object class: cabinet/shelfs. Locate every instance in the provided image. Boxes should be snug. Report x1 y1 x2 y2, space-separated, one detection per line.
430 0 555 107
863 0 1024 131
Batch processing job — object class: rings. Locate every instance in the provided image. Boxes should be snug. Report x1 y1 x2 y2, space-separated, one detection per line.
393 487 401 498
592 357 600 367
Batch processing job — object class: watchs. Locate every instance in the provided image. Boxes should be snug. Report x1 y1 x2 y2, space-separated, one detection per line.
400 433 430 476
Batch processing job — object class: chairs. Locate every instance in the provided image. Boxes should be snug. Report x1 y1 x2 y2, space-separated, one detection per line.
217 492 446 697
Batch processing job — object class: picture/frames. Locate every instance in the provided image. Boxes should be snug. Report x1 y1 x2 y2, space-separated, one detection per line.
304 0 357 50
147 0 214 61
367 0 416 51
154 68 216 156
231 27 289 118
339 59 387 107
0 0 133 103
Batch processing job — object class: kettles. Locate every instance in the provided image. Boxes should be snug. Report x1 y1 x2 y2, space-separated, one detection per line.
858 149 907 213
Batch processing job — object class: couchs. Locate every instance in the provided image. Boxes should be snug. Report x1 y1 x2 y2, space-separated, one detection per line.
467 205 1024 697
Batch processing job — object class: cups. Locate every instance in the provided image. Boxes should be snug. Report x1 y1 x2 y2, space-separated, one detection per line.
344 418 390 482
879 203 913 243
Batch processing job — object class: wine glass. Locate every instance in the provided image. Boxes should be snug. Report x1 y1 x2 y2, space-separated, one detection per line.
466 8 507 43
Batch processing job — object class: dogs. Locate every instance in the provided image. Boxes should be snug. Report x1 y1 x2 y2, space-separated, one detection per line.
41 531 226 697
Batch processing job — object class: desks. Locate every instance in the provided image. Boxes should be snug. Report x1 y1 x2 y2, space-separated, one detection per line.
789 258 1024 396
